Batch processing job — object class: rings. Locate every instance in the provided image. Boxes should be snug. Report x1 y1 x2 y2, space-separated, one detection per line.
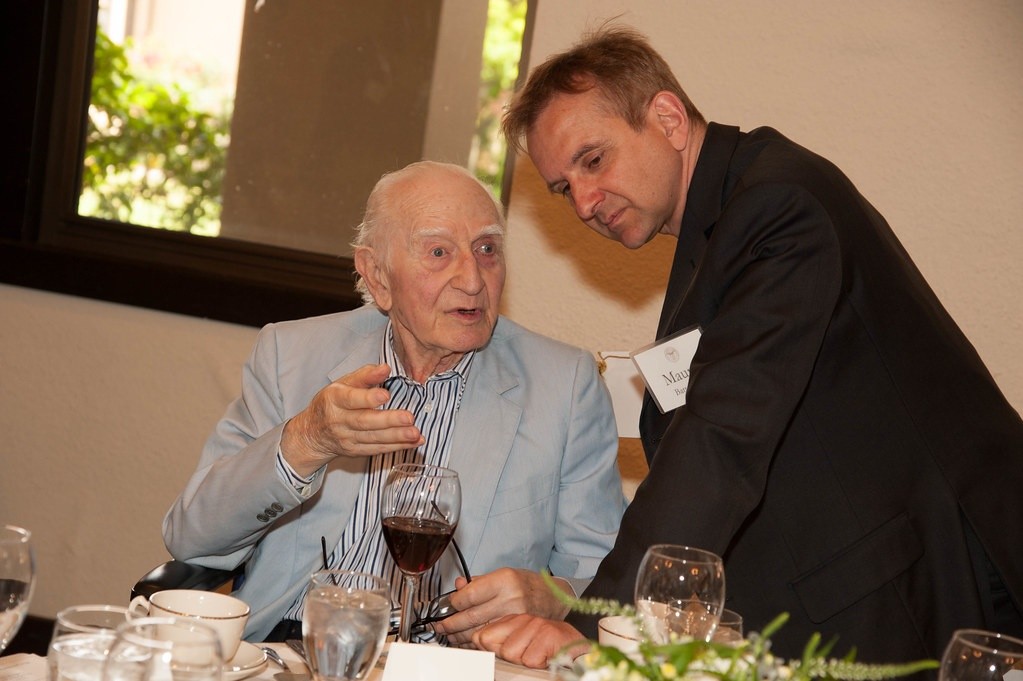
484 620 489 627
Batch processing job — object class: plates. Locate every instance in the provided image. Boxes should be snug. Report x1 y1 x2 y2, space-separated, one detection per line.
221 641 267 681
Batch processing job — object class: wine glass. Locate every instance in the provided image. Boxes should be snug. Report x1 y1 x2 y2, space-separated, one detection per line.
381 464 461 643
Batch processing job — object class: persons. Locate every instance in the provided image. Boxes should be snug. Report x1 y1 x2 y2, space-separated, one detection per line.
470 13 1023 681
161 160 627 649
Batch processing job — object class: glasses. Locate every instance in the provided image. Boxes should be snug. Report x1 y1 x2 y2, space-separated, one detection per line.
321 500 472 635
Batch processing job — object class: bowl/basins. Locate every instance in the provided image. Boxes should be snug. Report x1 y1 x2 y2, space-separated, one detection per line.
598 616 668 665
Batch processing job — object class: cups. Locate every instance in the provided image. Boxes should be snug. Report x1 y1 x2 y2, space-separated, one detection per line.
302 571 391 681
633 545 743 643
938 630 1023 681
130 589 251 666
0 524 35 656
0 604 221 681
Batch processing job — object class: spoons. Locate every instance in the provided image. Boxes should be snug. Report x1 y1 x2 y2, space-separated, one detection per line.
262 646 313 681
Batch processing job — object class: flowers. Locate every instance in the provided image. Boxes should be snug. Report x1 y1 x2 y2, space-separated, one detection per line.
541 567 944 681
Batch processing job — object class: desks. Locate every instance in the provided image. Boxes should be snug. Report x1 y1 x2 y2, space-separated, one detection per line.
0 628 586 681
